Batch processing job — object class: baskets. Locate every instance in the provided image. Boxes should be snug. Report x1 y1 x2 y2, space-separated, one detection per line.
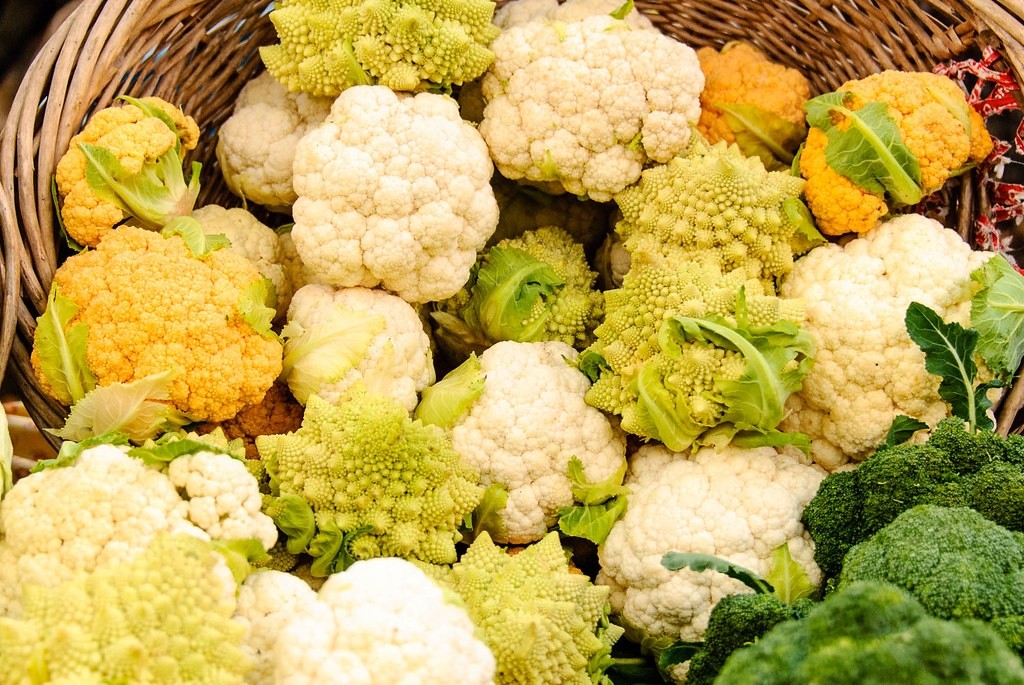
0 0 1023 488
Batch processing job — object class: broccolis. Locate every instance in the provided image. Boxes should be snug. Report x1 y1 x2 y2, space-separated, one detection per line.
0 0 1024 685
